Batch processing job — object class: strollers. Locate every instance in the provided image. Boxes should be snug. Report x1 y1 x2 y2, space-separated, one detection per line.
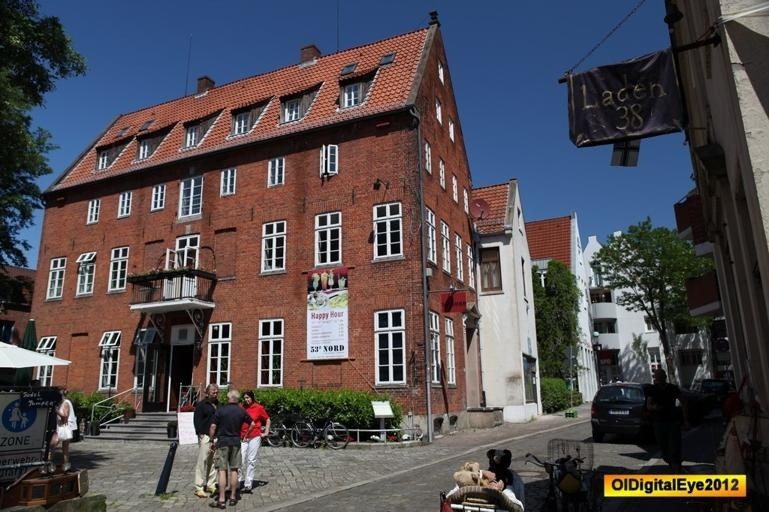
436 445 527 512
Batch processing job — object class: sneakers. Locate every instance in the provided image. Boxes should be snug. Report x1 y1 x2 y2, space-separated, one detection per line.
194 489 208 499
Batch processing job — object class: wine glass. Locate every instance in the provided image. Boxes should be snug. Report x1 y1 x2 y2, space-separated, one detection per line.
311 272 347 293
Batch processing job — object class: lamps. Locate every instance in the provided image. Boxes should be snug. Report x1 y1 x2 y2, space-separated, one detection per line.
39 462 57 478
61 462 71 474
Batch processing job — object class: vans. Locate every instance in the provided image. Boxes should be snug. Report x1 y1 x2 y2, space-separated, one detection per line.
588 382 656 439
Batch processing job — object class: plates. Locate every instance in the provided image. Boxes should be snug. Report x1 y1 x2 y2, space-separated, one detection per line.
308 292 326 308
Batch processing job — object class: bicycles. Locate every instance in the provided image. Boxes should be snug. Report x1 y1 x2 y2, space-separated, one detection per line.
262 400 352 451
522 436 596 511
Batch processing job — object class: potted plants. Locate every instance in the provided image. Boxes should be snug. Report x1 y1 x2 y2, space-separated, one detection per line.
116 400 136 419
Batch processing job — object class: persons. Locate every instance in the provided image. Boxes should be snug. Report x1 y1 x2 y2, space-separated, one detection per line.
193 383 224 498
208 390 256 509
238 390 272 491
47 395 79 471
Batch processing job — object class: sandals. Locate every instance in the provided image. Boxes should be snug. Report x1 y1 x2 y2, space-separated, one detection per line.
209 498 238 509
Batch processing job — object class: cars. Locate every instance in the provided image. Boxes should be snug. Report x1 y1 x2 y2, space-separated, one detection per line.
690 375 736 399
